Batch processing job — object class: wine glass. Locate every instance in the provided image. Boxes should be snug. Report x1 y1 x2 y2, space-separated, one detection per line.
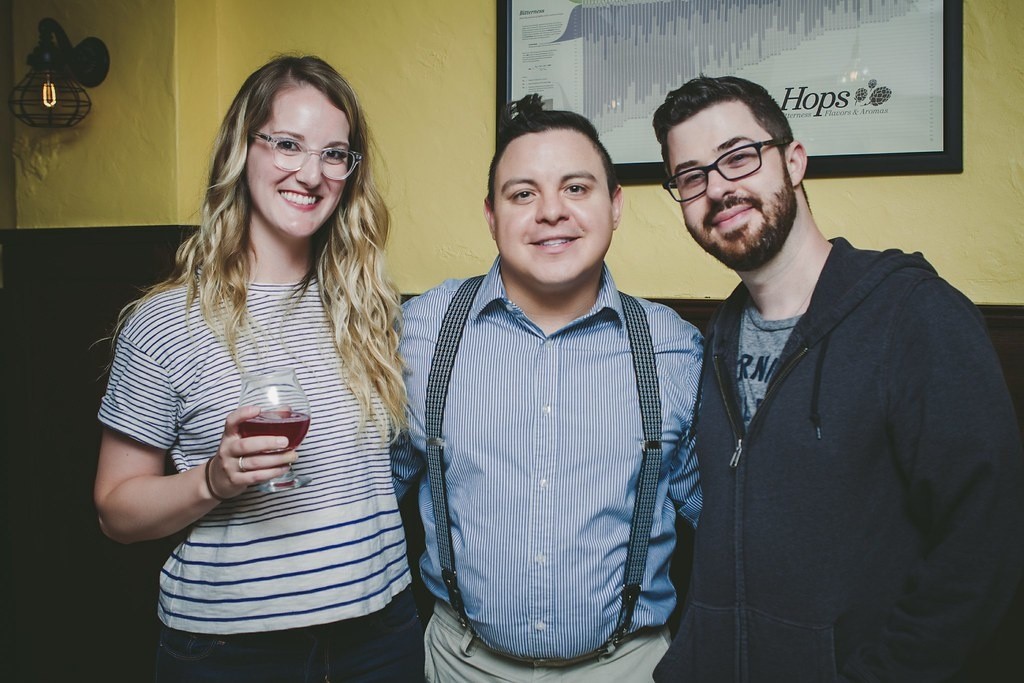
236 368 311 493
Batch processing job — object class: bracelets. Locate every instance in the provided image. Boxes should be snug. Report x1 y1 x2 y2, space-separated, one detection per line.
205 455 234 502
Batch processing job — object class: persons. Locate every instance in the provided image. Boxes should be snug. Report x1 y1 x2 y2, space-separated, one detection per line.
87 53 427 683
654 72 1024 683
389 96 704 683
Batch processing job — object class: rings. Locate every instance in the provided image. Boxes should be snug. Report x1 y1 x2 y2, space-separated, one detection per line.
238 456 246 471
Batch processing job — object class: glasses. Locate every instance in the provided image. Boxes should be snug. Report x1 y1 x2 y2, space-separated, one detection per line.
661 137 797 203
249 130 364 180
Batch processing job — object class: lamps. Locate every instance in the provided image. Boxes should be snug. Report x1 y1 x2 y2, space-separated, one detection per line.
9 17 111 129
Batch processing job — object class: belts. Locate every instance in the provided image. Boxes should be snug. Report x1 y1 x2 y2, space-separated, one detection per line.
473 628 646 668
